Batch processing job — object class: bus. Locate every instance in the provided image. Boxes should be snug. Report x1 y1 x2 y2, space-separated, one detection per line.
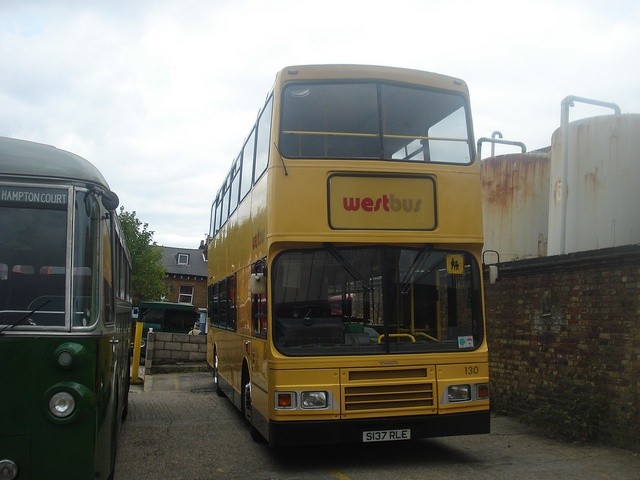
0 136 133 480
205 65 500 445
130 301 196 360
